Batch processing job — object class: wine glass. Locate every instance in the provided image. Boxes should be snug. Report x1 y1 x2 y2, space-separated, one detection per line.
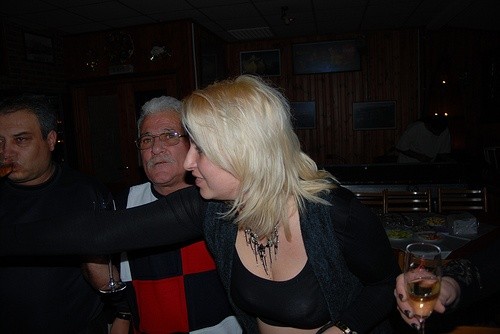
404 243 442 333
90 197 127 293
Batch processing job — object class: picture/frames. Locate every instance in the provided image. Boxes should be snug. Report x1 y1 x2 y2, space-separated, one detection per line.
240 49 282 77
288 100 317 130
352 100 397 131
291 38 362 76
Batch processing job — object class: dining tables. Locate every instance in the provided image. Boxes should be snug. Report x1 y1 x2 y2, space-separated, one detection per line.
383 212 497 270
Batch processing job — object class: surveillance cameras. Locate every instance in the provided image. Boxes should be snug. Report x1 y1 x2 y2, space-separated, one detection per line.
281 6 296 25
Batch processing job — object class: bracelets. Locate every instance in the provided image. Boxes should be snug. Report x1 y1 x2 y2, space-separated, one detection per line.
113 312 132 320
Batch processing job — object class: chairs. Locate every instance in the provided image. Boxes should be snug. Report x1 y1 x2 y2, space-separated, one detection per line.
352 187 488 217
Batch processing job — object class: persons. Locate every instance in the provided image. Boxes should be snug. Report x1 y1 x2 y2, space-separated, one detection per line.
82 94 249 334
394 233 500 334
0 99 132 334
0 74 402 334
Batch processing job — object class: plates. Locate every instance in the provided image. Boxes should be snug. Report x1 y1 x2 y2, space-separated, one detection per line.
387 215 448 242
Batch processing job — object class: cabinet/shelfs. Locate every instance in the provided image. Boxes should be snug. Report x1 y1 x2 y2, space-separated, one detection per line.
63 18 228 182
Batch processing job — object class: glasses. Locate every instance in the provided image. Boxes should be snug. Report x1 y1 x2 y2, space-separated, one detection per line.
134 131 188 149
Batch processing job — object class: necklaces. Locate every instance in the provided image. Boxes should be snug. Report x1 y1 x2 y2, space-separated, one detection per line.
244 220 282 279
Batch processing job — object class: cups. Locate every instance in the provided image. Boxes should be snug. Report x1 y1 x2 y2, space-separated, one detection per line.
0 152 15 178
